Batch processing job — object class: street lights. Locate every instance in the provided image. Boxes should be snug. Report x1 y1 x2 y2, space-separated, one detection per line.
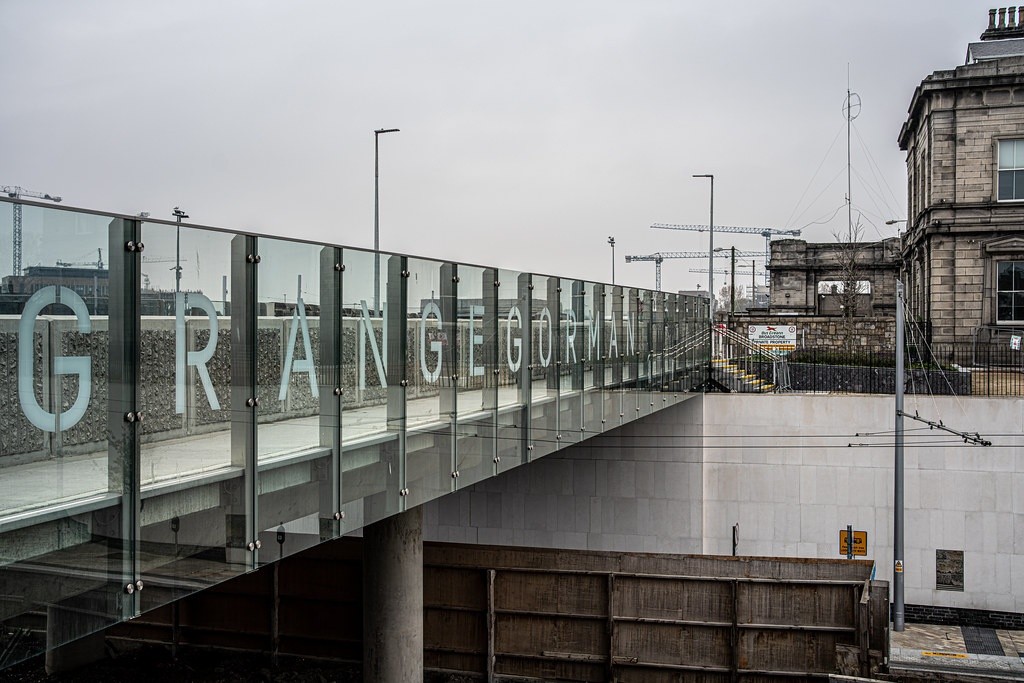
173 208 191 316
608 235 617 284
690 173 715 322
372 127 402 315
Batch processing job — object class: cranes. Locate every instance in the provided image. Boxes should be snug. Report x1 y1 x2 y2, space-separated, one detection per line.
621 223 801 307
0 185 64 277
54 247 189 271
